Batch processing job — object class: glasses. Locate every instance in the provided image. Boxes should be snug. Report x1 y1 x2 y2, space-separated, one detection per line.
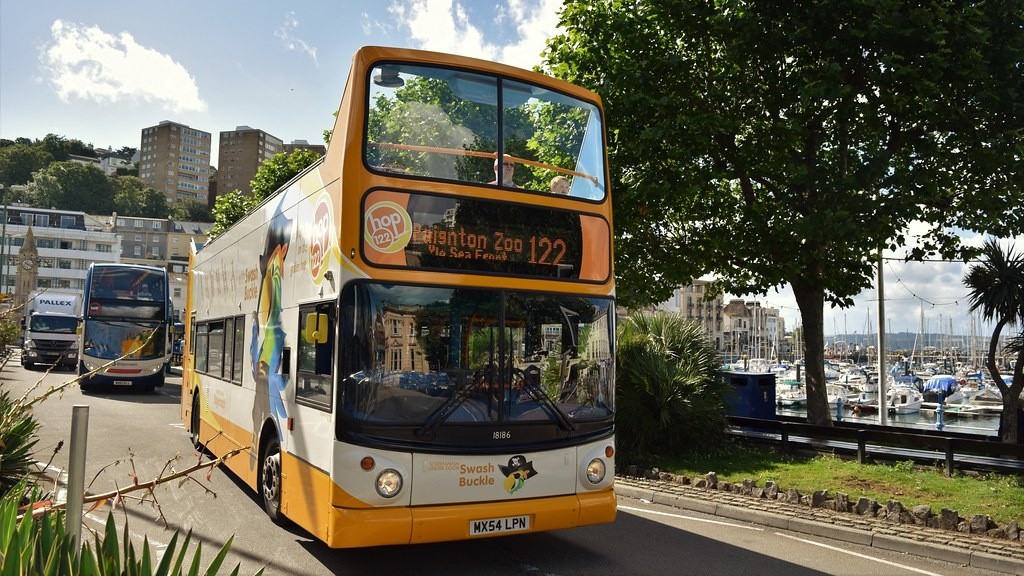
503 162 515 167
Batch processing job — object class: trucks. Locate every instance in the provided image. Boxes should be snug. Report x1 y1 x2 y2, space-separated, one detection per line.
20 293 83 372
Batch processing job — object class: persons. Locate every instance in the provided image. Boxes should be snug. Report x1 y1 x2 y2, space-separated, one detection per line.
134 335 142 341
550 176 570 195
136 283 153 302
486 154 520 189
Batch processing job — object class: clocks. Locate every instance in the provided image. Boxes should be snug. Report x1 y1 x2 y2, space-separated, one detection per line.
21 259 34 270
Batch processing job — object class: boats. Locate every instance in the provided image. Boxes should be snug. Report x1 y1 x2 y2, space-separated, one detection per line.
717 297 1024 420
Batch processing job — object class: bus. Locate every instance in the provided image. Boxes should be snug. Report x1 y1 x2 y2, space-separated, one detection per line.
164 297 174 374
77 262 169 395
180 45 618 549
173 323 185 355
